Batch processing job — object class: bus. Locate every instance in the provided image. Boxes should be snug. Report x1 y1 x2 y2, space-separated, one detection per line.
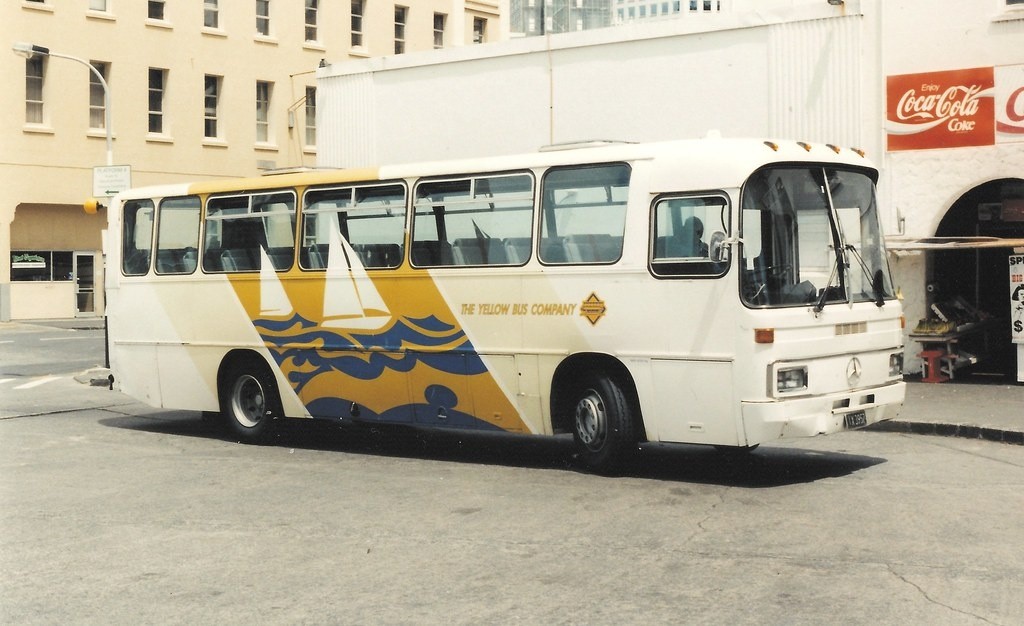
100 136 907 473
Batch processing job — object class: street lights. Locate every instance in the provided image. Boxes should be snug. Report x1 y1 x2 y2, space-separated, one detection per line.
11 45 116 318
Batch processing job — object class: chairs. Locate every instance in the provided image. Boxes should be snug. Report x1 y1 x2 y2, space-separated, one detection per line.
125 233 678 277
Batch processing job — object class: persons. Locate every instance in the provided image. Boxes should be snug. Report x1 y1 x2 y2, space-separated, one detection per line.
673 217 710 258
